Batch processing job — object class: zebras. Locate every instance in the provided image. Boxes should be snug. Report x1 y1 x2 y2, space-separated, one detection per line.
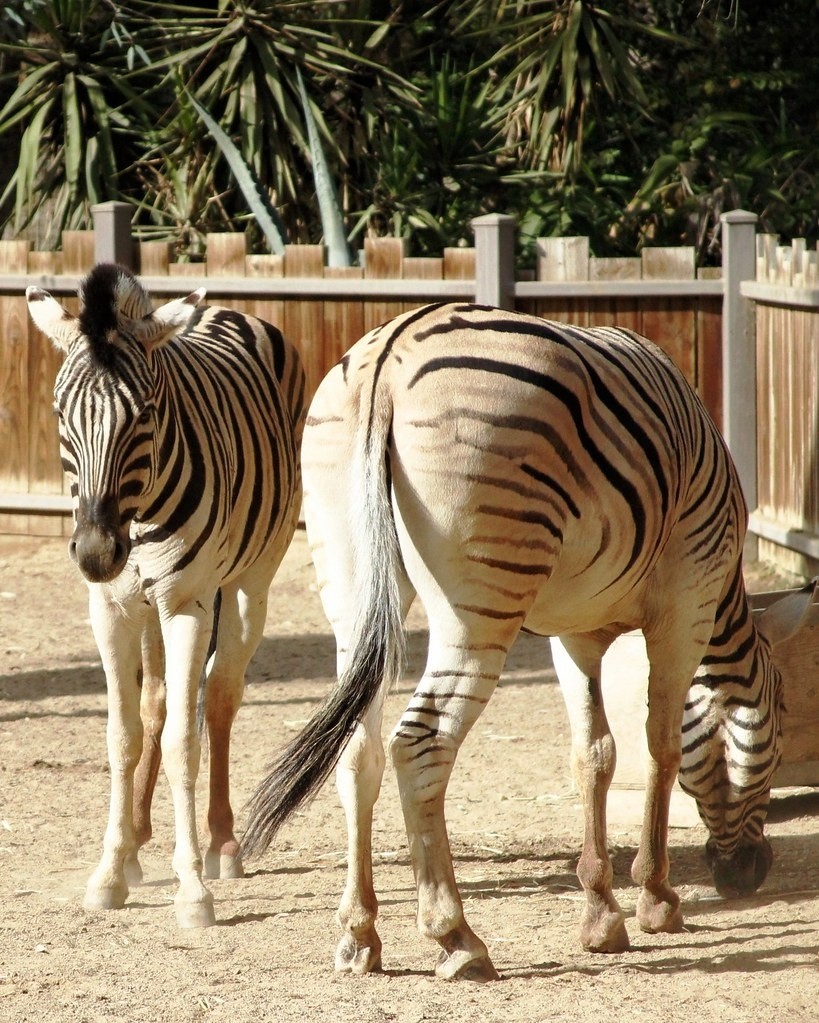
230 301 819 984
25 262 308 928
